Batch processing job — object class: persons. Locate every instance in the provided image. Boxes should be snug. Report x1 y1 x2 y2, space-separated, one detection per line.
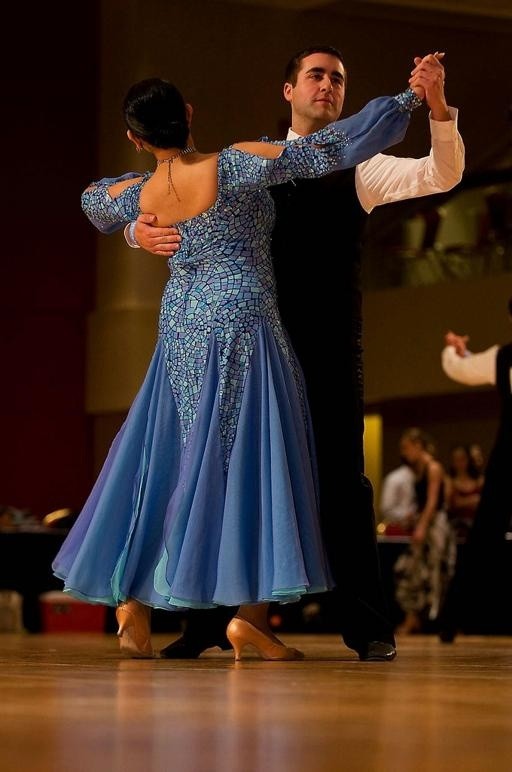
75 52 448 671
443 329 473 357
122 47 470 663
369 425 497 636
438 313 511 637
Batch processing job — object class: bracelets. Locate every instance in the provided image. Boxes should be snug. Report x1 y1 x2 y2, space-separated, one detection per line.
129 221 139 247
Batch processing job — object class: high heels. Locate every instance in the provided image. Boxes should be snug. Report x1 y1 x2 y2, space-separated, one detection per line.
225 614 305 662
115 597 154 659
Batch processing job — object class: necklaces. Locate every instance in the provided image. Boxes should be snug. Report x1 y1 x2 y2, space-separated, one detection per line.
155 143 200 203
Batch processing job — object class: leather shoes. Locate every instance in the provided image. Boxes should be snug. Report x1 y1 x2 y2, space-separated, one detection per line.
359 640 397 661
159 629 233 660
